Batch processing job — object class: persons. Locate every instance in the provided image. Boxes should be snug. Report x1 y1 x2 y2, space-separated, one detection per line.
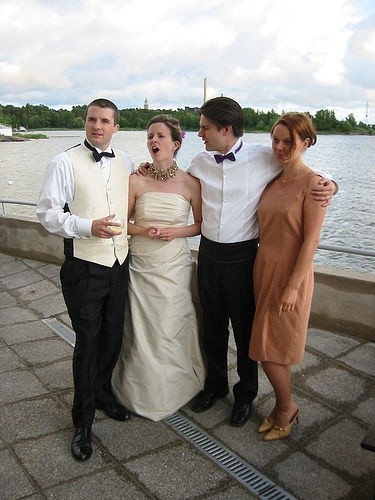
249 111 326 441
133 96 338 427
111 114 207 422
36 99 138 461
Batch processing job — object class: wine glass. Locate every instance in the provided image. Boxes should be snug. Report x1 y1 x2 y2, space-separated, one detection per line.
106 213 124 248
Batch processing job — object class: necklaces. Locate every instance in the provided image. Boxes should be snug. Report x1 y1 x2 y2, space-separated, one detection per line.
148 160 178 182
278 172 299 184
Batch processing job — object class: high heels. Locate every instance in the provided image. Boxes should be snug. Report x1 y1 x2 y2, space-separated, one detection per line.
257 414 275 433
263 409 301 441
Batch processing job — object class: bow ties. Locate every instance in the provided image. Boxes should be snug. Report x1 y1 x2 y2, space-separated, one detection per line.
214 140 243 164
84 138 115 162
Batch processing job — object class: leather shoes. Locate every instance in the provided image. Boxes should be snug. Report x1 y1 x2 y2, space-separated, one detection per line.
230 400 252 426
192 386 229 413
95 400 130 421
71 427 92 462
